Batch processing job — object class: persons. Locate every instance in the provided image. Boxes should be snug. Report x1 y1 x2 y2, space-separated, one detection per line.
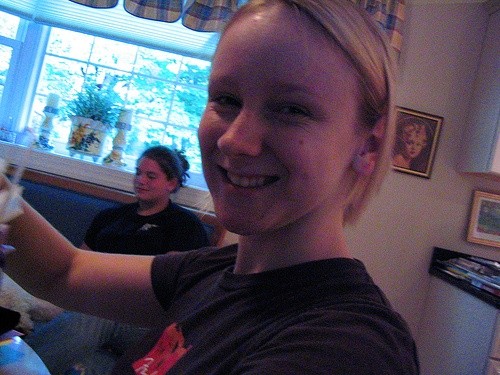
0 0 424 374
395 121 429 169
75 143 209 256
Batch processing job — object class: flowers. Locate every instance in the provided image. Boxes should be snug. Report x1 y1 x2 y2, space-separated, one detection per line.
62 64 127 130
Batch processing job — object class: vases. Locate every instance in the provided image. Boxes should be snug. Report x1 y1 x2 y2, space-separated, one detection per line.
66 116 108 161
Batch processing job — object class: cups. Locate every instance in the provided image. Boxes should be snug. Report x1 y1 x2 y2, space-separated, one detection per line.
0 128 33 224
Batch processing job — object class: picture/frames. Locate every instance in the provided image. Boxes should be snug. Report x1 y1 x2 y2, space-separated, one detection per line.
392 105 444 180
465 191 500 248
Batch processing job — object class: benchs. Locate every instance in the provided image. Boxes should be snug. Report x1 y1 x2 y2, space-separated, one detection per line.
0 161 227 338
